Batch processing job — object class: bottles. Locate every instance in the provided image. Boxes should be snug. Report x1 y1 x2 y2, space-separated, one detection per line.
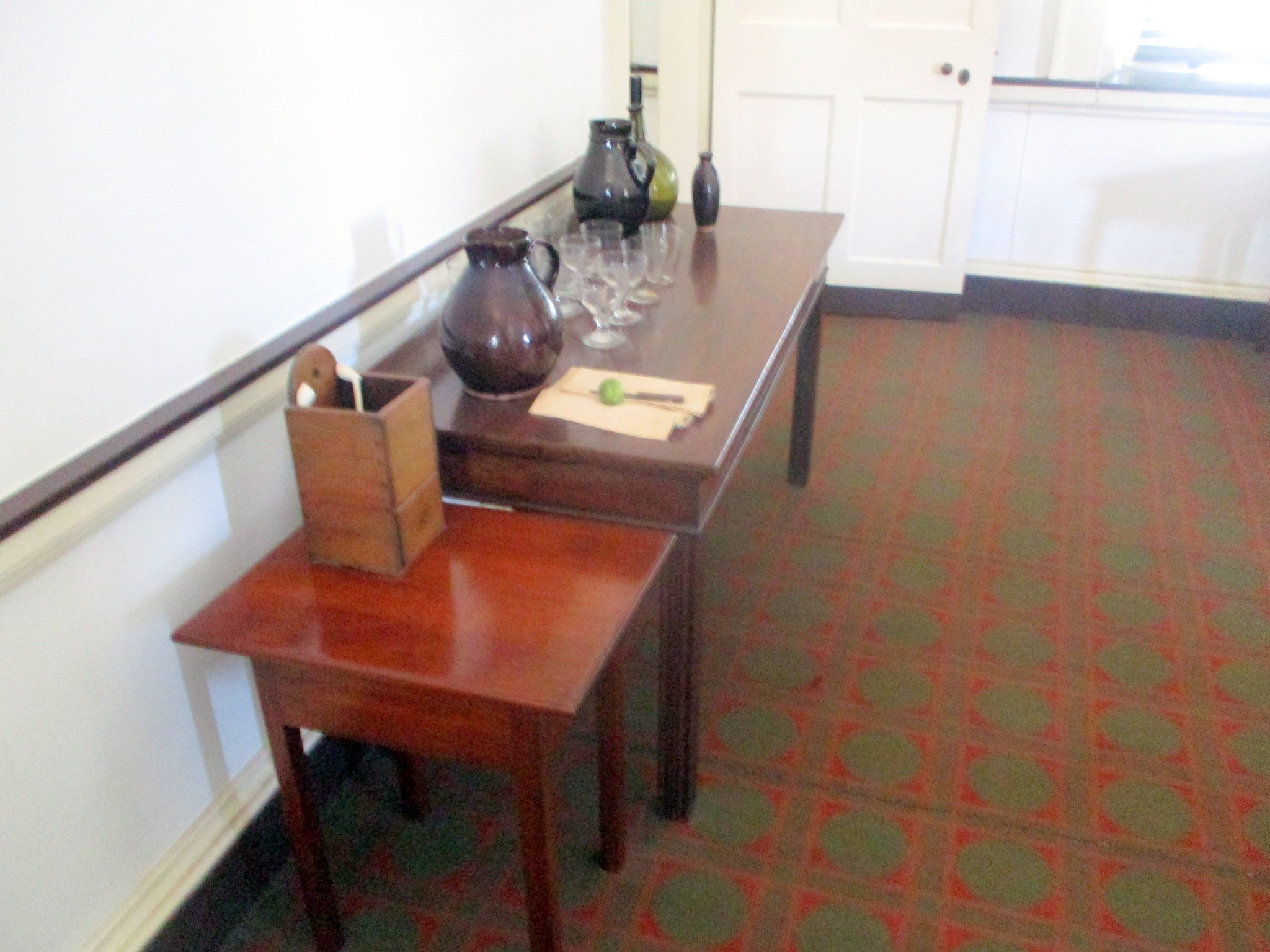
626 104 677 220
690 153 720 232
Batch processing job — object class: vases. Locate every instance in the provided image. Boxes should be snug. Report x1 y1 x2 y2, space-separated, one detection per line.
431 226 564 401
570 77 680 241
693 151 722 234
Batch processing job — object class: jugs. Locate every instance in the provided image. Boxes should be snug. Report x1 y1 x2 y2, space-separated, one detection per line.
438 227 563 401
571 120 657 236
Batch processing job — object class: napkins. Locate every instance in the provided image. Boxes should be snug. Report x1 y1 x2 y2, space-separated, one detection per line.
528 366 715 441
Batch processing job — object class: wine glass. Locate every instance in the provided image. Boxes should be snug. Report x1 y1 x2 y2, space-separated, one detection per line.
523 235 648 347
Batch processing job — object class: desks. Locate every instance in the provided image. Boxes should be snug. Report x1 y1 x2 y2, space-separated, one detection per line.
362 202 844 822
171 501 678 952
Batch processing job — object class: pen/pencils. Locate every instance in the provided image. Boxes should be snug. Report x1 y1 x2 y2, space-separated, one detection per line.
590 391 683 403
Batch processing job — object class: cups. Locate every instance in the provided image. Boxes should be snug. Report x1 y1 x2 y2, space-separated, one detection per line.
579 219 681 304
